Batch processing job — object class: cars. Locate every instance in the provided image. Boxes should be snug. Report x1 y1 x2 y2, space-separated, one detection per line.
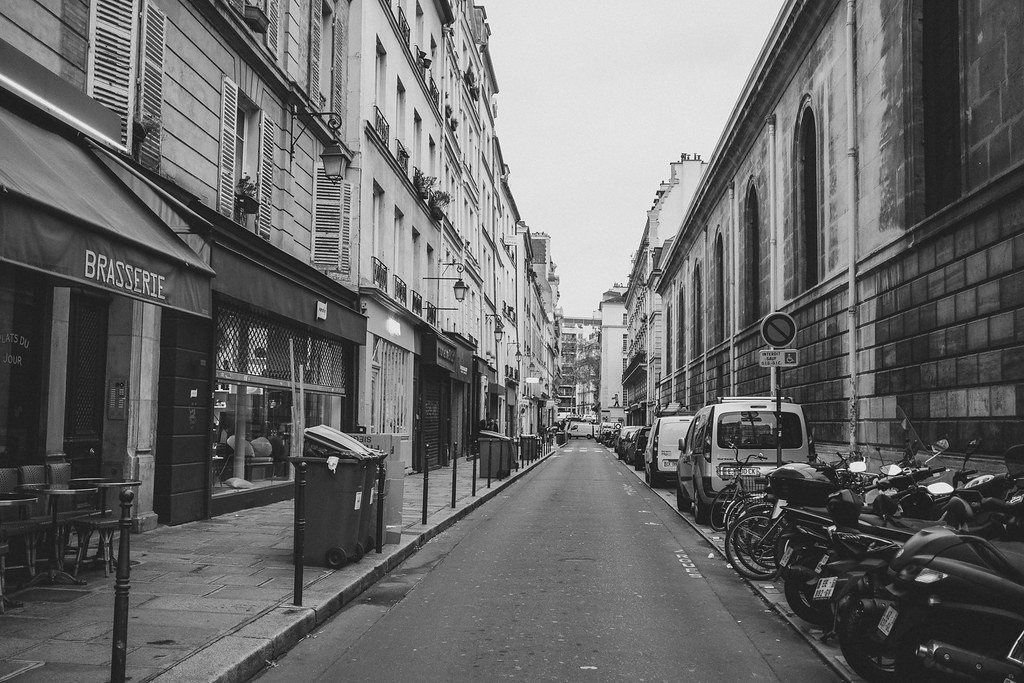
617 424 651 471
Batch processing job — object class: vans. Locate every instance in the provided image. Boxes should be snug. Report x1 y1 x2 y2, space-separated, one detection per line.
676 394 815 523
643 402 696 489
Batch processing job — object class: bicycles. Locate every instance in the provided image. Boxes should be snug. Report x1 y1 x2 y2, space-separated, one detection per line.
700 441 873 580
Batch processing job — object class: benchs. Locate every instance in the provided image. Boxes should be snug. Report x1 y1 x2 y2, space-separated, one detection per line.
244 457 274 479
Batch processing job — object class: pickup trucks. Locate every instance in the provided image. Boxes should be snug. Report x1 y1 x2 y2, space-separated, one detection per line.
581 415 595 423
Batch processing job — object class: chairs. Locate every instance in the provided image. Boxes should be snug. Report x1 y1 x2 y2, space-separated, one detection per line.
46 462 116 579
0 468 39 604
20 463 75 574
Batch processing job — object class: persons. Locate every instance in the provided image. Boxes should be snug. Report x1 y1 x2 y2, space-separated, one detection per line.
487 418 498 432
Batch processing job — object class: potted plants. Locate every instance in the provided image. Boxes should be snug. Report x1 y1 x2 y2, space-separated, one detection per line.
471 86 481 102
237 170 263 214
463 67 475 86
133 105 168 144
245 4 271 33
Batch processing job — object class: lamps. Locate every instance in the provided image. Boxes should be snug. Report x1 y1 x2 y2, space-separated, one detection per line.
413 171 441 199
507 342 522 362
539 376 544 385
485 314 504 343
423 277 468 302
431 191 454 220
291 104 352 185
545 383 549 389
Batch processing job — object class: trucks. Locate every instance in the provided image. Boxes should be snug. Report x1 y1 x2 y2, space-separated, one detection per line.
567 422 614 438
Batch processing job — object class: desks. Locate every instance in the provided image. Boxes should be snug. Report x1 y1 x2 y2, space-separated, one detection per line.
16 482 98 586
0 494 39 615
70 477 143 569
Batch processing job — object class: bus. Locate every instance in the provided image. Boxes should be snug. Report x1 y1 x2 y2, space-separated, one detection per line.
566 414 581 422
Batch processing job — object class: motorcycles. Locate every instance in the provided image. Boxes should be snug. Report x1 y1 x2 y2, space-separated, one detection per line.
766 435 1024 683
601 430 617 447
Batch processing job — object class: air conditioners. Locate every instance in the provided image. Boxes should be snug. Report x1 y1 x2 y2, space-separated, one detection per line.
529 362 535 373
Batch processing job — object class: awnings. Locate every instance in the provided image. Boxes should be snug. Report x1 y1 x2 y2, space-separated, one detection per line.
0 107 215 319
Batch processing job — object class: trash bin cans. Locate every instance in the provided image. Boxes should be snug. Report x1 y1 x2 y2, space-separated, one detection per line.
478 430 512 478
556 432 565 444
288 424 389 568
521 433 537 460
